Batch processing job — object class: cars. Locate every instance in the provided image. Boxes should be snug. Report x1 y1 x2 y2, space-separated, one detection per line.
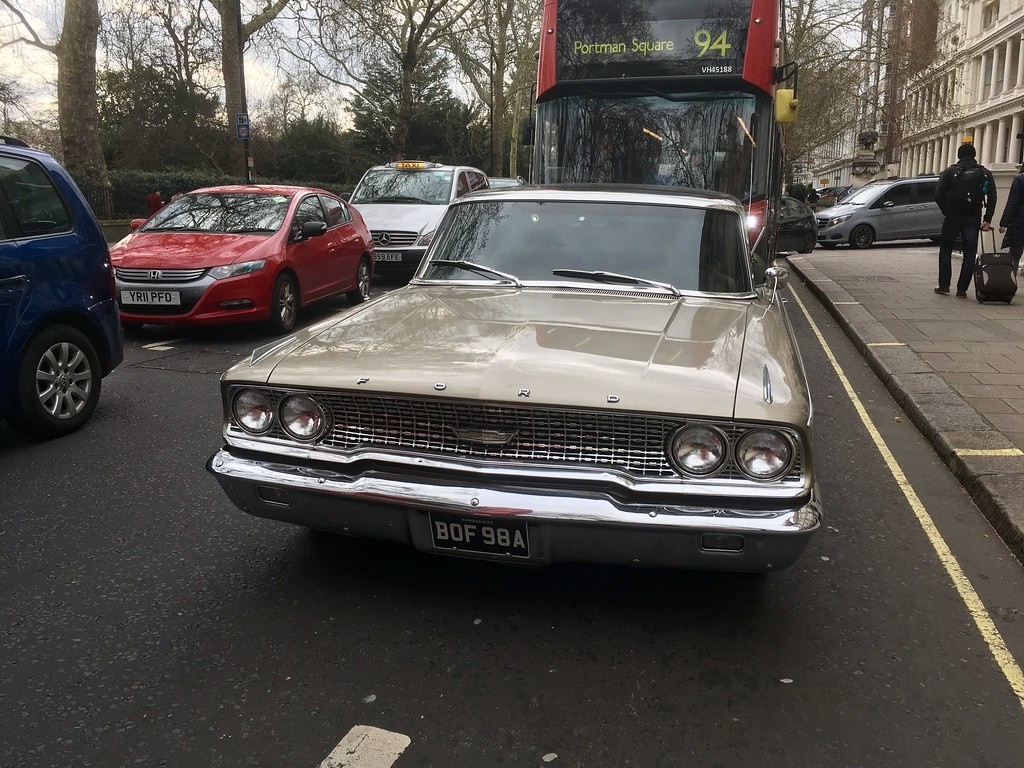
776 196 819 254
206 178 825 576
486 174 528 189
109 186 373 338
812 175 963 250
807 183 857 210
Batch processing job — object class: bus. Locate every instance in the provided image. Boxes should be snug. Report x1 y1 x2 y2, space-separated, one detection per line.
521 0 801 280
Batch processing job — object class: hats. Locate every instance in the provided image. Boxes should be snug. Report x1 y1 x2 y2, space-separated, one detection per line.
958 141 976 159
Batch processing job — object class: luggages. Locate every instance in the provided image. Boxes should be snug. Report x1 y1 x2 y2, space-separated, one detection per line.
974 226 1018 304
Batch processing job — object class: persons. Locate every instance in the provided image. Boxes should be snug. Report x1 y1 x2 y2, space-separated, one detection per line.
148 184 187 218
933 144 997 299
783 185 820 212
999 162 1024 268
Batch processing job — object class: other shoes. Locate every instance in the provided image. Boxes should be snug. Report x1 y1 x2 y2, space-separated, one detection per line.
935 286 950 295
957 291 967 299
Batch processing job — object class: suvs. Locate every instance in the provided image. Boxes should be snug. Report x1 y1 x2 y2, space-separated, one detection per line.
1 134 125 440
339 159 491 273
